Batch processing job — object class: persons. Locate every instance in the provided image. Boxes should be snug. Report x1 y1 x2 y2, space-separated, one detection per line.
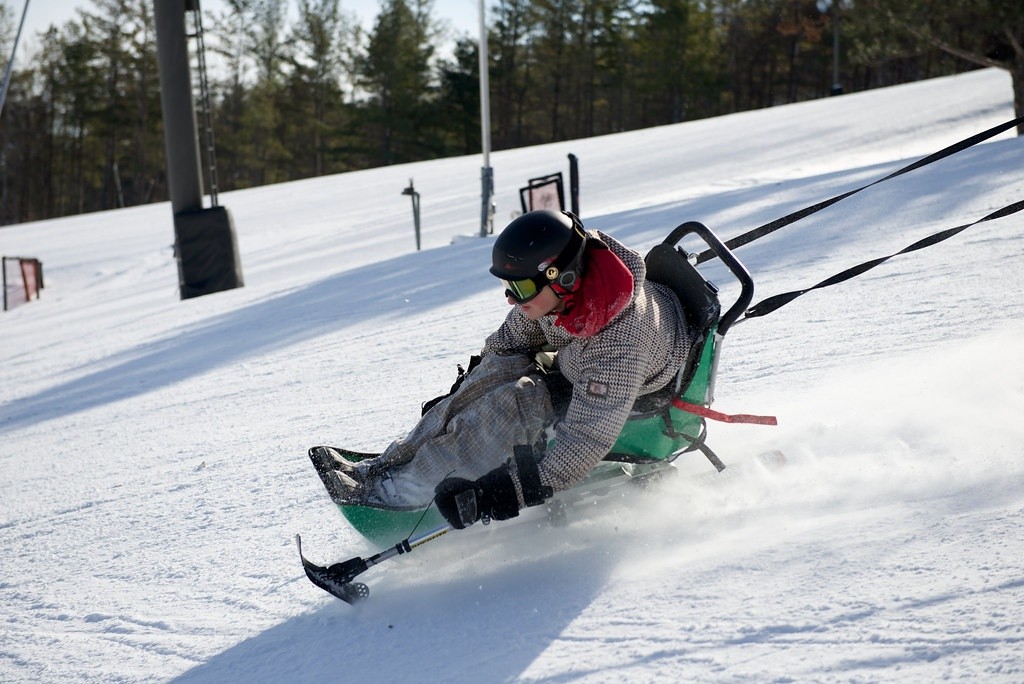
319 208 695 529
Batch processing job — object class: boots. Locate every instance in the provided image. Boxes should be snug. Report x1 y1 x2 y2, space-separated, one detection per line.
315 437 402 471
325 469 412 509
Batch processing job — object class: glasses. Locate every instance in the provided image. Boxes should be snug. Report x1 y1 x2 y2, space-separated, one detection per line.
499 276 543 304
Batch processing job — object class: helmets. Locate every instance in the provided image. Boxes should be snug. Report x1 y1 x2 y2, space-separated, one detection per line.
489 210 586 281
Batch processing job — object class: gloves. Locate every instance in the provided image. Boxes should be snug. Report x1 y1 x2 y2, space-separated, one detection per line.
434 462 519 529
421 355 483 417
